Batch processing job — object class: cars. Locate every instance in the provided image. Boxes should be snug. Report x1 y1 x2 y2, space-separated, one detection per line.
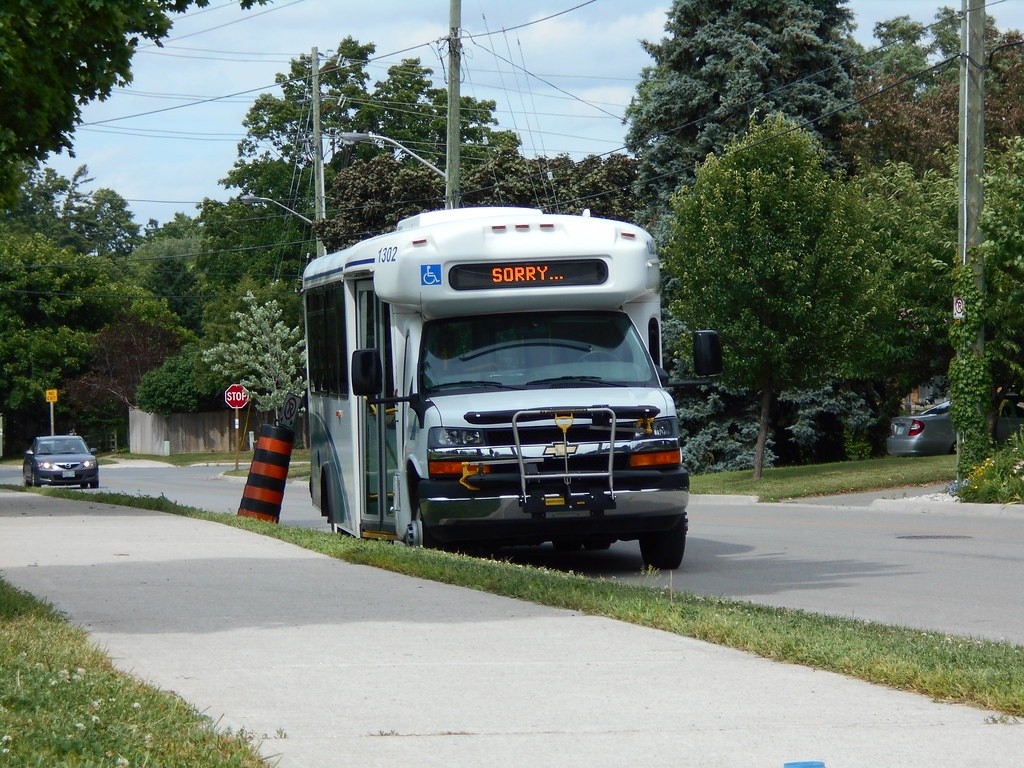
885 398 1024 458
23 435 99 490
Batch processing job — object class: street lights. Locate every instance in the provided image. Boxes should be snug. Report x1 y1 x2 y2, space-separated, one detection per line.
338 130 459 209
240 194 327 259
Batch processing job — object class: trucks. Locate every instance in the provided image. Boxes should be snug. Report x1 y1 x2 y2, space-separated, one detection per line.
300 206 724 571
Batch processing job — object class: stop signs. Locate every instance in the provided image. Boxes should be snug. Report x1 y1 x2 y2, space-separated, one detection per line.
225 384 251 409
